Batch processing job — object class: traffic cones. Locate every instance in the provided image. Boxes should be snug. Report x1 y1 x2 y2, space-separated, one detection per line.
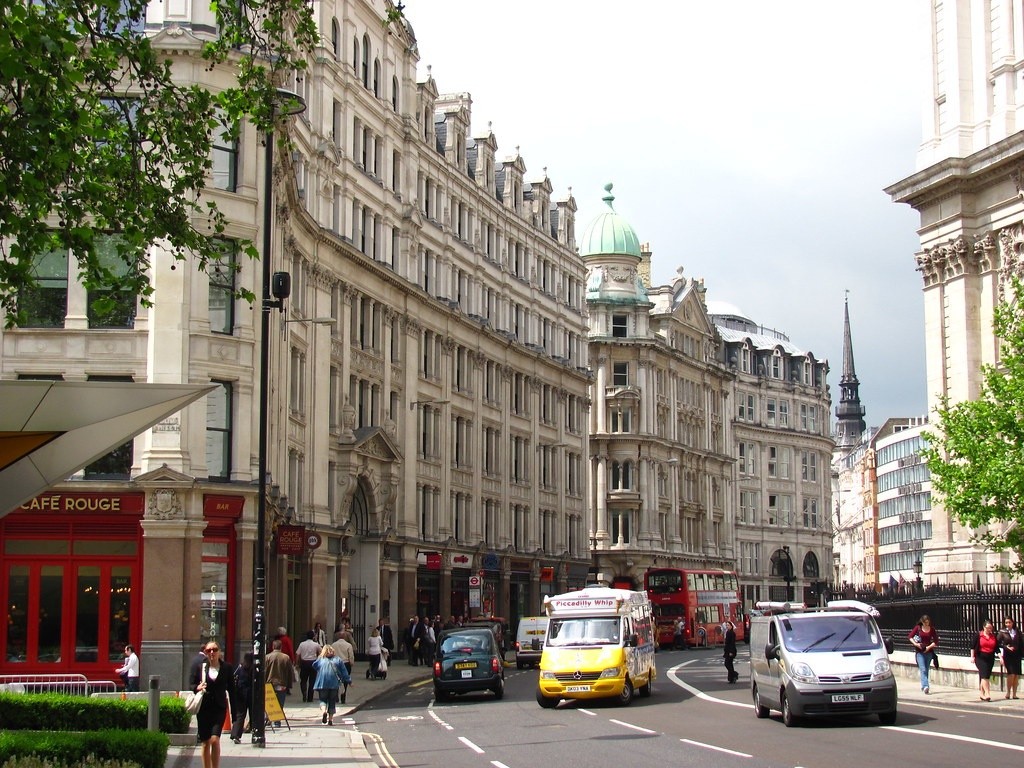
220 700 232 734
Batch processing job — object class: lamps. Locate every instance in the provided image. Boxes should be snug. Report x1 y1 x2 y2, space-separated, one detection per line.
913 556 922 578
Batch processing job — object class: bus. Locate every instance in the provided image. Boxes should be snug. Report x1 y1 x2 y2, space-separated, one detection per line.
643 567 745 647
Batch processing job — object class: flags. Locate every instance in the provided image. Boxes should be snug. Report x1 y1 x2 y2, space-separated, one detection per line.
898 574 906 594
890 573 898 590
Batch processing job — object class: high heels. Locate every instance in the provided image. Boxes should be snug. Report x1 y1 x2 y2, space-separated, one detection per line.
980 697 991 702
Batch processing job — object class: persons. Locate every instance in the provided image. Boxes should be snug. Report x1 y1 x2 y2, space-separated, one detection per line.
264 641 293 728
745 614 751 643
230 651 255 744
312 623 327 648
311 644 349 725
188 643 208 743
720 615 737 652
909 614 939 695
270 626 295 696
191 640 238 768
376 617 395 667
723 621 739 685
997 616 1023 699
653 624 659 653
670 616 686 652
110 628 128 653
969 619 1004 701
365 629 384 681
329 616 358 704
115 644 140 692
403 611 470 668
296 630 322 703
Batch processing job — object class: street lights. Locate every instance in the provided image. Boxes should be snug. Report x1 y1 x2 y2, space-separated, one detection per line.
230 85 306 747
912 556 923 594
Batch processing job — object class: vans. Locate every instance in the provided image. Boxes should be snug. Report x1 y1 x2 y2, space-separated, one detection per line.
749 606 898 728
514 616 551 668
536 587 657 709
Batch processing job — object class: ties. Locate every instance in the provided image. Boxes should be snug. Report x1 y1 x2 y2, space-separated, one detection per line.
380 626 383 639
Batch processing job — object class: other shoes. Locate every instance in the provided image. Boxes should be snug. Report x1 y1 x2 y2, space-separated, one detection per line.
1013 698 1019 699
1005 696 1010 699
233 737 241 744
323 712 327 723
275 723 281 727
329 721 332 725
924 687 929 694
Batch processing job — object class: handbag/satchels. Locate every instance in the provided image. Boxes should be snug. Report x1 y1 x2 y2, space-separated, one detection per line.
185 663 207 715
338 682 345 694
914 628 922 643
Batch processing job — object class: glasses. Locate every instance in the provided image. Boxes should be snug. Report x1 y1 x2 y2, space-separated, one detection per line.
205 647 219 653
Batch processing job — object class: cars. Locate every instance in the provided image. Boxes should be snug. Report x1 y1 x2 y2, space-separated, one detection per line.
431 616 510 703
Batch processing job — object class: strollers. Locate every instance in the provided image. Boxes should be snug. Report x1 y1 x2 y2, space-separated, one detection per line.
366 649 390 681
